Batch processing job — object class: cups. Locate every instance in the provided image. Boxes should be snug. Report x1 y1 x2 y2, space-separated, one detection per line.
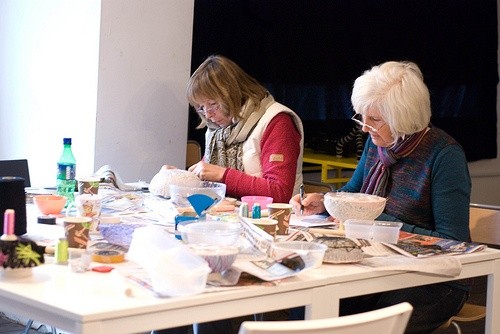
253 218 278 239
64 216 92 248
78 180 99 196
266 203 293 234
78 194 107 232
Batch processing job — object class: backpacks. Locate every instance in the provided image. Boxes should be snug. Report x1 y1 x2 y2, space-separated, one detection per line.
310 129 354 156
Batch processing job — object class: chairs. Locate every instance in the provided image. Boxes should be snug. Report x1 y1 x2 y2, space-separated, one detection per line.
186 139 201 169
238 302 414 334
440 202 500 334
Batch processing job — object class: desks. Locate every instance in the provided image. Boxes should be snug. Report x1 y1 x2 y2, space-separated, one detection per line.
0 199 500 334
302 148 360 183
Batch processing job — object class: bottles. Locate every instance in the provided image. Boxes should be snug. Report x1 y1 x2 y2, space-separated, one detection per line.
57 237 68 264
56 138 76 207
0 207 17 241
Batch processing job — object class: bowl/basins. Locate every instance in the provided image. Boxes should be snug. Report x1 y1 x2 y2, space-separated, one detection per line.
35 194 67 215
323 192 387 220
343 219 403 246
169 180 226 213
241 196 273 213
273 241 329 269
176 219 244 283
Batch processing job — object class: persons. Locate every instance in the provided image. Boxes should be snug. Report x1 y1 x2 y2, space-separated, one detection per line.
252 61 476 334
157 55 305 334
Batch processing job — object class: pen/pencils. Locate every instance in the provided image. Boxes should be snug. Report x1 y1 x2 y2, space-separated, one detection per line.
126 187 149 192
299 183 305 215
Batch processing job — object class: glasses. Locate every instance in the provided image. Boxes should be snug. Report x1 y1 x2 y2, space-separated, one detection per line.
351 113 389 136
196 103 221 118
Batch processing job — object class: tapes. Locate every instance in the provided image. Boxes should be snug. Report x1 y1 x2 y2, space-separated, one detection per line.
91 249 125 264
37 217 56 225
